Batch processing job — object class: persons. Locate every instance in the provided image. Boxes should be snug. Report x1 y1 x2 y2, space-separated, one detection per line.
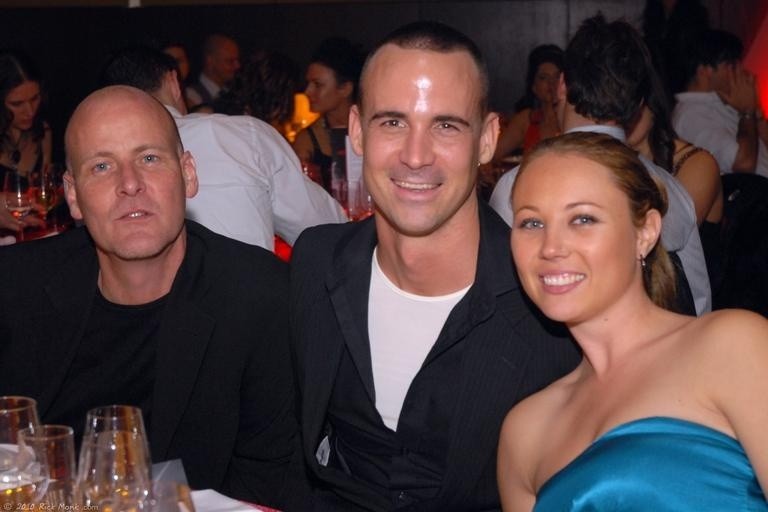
0 1 768 511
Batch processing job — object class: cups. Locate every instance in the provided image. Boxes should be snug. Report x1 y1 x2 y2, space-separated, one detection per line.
0 395 154 511
331 175 346 202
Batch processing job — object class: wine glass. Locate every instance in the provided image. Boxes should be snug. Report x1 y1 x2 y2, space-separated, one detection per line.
362 176 376 223
1 166 65 246
